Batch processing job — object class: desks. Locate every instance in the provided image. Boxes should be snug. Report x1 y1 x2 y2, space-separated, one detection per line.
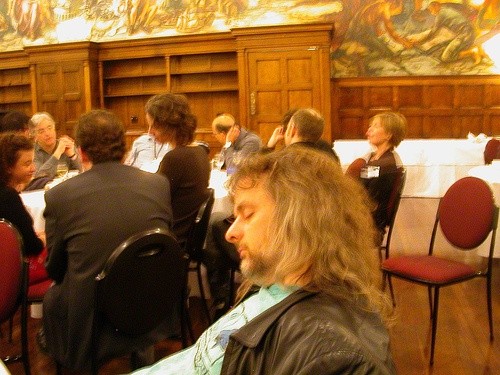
333 139 500 259
17 170 235 318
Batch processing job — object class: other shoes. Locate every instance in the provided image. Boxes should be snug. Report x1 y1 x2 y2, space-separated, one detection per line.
36 327 50 354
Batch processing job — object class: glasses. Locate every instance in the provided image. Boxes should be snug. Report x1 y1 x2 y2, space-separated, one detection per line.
212 132 223 138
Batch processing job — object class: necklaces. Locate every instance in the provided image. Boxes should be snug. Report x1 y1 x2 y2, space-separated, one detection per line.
154 139 164 160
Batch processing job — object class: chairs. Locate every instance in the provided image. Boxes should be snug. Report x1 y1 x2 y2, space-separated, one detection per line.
185 187 212 345
55 226 190 375
378 165 407 305
0 218 53 375
381 177 499 366
344 159 368 181
483 138 500 165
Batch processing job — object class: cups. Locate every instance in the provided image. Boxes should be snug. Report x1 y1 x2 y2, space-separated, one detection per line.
57 162 68 178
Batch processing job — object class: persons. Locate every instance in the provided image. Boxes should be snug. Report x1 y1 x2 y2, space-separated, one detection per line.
126 145 401 375
0 111 33 140
355 113 406 247
24 113 82 190
145 92 211 251
260 107 340 162
0 133 45 256
212 115 262 171
36 111 174 375
123 134 171 173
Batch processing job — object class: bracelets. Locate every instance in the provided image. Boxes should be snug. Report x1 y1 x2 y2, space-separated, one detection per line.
69 154 75 158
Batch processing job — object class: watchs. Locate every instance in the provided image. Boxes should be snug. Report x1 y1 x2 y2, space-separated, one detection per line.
225 141 234 148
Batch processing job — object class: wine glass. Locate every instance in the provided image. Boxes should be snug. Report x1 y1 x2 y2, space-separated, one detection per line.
214 154 224 172
